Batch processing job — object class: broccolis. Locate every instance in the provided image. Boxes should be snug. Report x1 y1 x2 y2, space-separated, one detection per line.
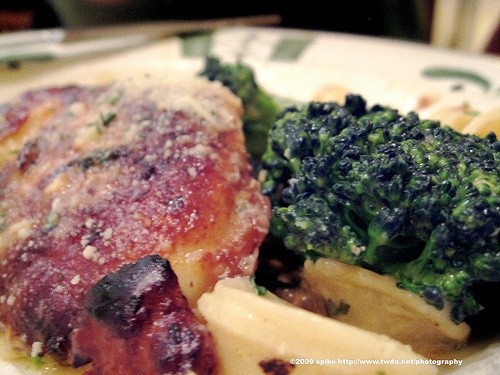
263 96 500 324
203 55 278 153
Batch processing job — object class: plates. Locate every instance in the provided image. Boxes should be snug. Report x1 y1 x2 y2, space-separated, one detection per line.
1 26 500 142
